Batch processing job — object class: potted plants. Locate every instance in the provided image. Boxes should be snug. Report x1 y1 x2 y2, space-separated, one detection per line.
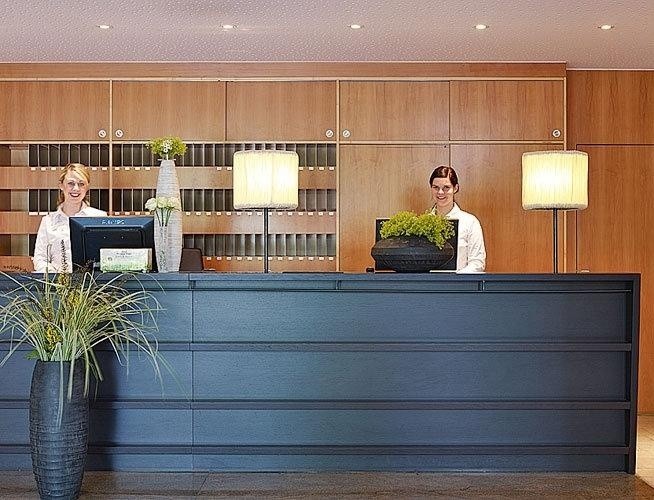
0 254 186 500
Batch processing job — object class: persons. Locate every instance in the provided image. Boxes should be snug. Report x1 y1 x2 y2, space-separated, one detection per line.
33 163 108 274
425 165 487 274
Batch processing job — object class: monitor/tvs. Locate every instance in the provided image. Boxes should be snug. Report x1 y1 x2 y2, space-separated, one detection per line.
374 217 458 273
69 215 153 274
